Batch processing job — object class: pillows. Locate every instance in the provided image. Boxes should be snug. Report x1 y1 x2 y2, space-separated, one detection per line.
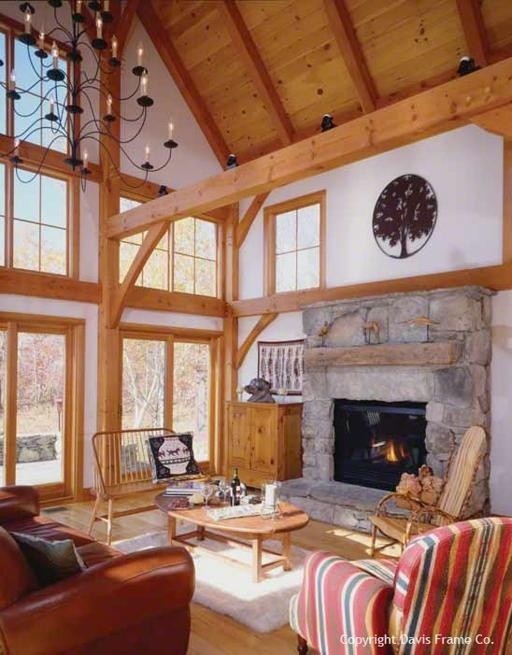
5 529 89 578
146 433 203 482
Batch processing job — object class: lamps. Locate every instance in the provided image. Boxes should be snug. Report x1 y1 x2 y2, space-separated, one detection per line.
1 0 178 196
225 153 238 168
321 113 336 131
457 56 481 78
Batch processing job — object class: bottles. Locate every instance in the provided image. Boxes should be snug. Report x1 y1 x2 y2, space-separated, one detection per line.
231 468 241 505
260 478 282 519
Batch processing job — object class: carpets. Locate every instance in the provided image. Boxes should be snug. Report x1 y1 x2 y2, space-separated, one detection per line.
106 523 317 635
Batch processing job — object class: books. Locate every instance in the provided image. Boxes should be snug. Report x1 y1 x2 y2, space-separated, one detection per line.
163 481 210 497
206 504 260 521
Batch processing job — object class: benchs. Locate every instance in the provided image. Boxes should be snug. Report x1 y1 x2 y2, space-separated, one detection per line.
90 427 208 542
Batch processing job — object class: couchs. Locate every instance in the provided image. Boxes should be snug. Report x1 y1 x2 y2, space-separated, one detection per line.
0 485 194 655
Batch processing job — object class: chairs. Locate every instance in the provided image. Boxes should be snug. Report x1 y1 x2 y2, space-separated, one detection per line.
290 515 512 655
367 423 487 558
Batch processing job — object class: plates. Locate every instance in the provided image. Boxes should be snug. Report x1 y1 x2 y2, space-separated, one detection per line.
168 496 193 510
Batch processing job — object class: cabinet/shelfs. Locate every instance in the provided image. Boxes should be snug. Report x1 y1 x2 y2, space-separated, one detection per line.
222 401 303 490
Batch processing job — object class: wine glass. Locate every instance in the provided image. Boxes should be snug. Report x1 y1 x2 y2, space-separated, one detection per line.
218 480 232 505
200 486 213 512
